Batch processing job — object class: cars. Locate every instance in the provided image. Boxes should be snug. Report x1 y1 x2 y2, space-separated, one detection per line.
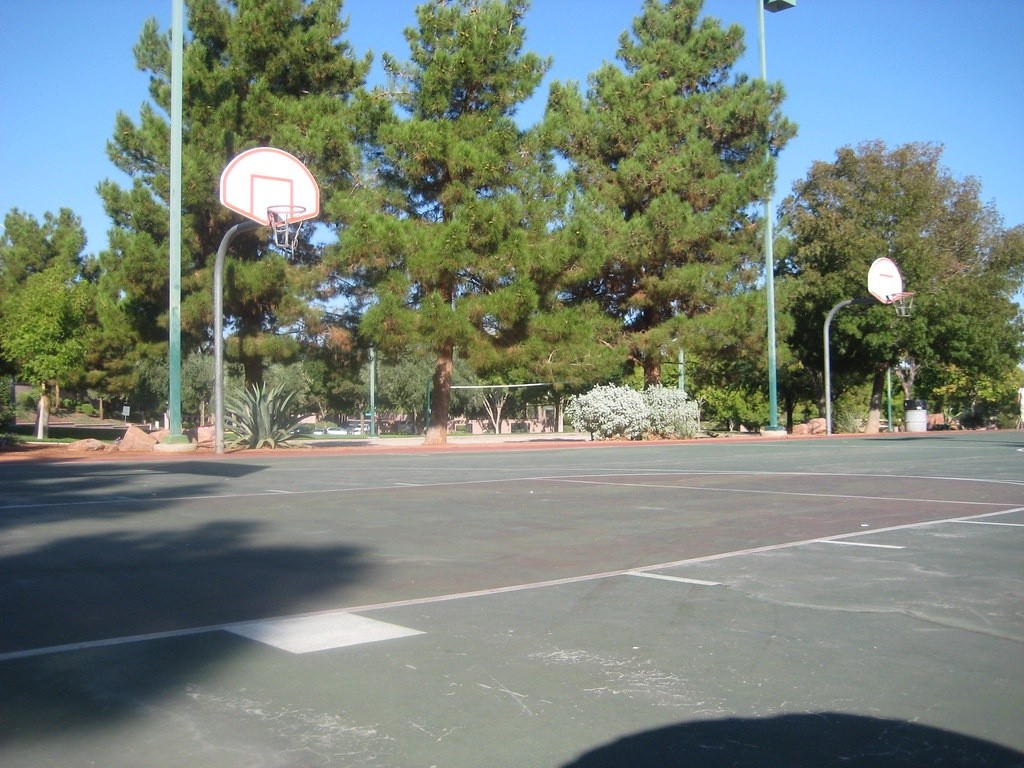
314 426 348 436
879 418 889 431
349 424 371 435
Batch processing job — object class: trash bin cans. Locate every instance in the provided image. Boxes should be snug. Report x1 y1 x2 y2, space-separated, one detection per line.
903 399 928 432
